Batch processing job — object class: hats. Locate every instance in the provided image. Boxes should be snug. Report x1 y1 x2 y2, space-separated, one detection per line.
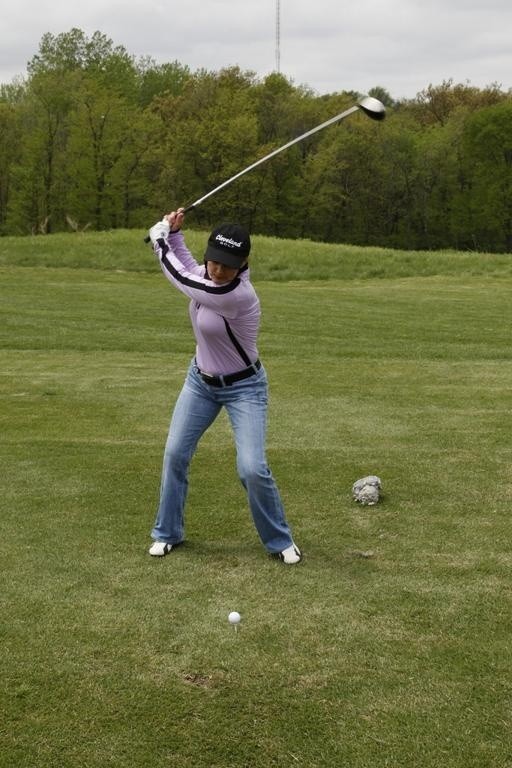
204 223 251 268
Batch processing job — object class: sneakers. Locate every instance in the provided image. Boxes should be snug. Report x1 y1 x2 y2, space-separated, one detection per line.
149 541 172 555
278 542 301 564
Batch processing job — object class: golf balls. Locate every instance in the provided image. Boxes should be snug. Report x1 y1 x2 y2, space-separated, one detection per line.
228 611 242 623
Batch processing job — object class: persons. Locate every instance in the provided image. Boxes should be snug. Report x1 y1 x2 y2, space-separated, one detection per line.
146 205 303 567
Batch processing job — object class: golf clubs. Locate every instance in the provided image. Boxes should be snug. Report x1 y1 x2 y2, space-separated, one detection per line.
143 98 386 244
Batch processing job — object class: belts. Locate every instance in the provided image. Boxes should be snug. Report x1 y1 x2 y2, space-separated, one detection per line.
194 357 261 388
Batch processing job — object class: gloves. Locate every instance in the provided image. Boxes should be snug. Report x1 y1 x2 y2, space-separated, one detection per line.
149 221 170 243
